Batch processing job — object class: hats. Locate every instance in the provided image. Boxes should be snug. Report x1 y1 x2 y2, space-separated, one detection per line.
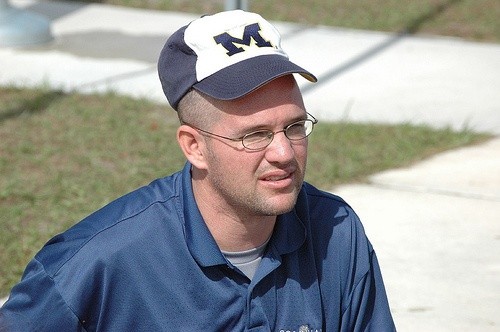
157 9 317 111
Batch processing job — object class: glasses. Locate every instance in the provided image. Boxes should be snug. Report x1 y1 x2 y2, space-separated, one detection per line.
181 111 318 151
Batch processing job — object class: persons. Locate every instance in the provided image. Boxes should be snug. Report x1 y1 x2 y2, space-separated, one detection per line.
0 9 396 332
